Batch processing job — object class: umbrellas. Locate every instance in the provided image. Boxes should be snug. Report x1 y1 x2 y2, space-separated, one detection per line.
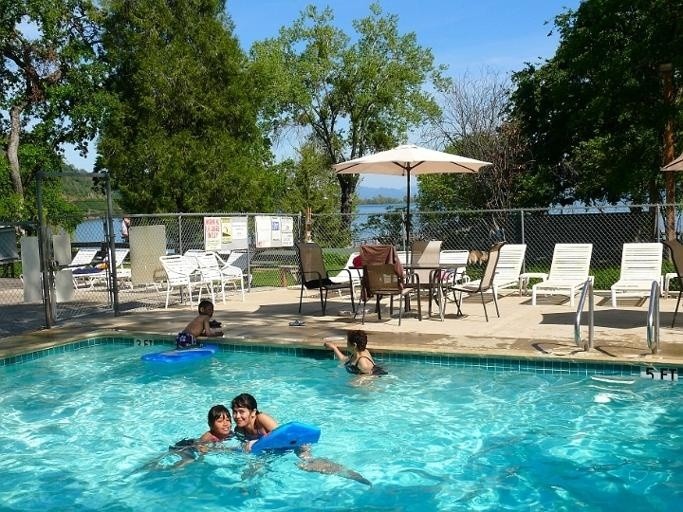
332 145 494 283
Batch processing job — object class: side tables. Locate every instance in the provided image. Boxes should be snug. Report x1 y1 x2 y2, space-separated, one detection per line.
665 273 681 299
519 272 548 296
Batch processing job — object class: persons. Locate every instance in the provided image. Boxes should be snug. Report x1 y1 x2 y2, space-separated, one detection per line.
151 404 231 475
175 300 225 348
324 331 375 385
231 392 372 489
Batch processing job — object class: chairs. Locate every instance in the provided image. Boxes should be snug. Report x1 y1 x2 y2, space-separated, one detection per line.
531 243 595 328
20 248 132 294
294 240 527 326
611 241 663 308
663 237 683 327
159 247 253 310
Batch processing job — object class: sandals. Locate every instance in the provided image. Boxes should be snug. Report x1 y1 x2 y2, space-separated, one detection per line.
289 319 306 326
